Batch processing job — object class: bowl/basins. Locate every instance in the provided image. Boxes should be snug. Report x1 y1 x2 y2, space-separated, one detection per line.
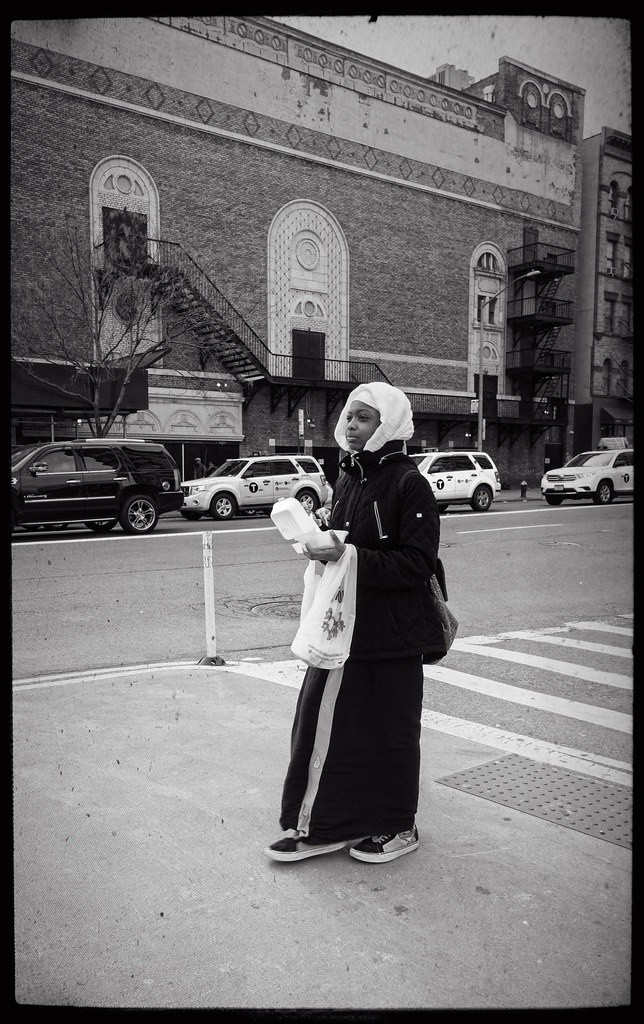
270 498 349 554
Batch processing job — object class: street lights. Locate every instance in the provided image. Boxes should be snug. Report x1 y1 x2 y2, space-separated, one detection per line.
478 270 542 452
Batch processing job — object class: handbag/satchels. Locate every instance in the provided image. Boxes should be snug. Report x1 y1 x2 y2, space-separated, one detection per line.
291 544 358 670
399 470 460 664
299 559 326 627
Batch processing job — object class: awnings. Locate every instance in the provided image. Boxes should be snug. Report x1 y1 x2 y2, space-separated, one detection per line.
601 407 634 424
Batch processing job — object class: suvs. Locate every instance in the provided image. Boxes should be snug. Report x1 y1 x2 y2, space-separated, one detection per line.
408 452 501 513
180 455 328 520
11 436 185 535
539 449 636 505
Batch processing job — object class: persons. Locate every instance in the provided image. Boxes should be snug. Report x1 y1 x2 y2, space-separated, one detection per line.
194 458 206 479
264 381 441 863
205 459 216 477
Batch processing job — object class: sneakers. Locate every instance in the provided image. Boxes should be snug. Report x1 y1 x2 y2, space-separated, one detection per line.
266 839 347 862
349 825 419 863
